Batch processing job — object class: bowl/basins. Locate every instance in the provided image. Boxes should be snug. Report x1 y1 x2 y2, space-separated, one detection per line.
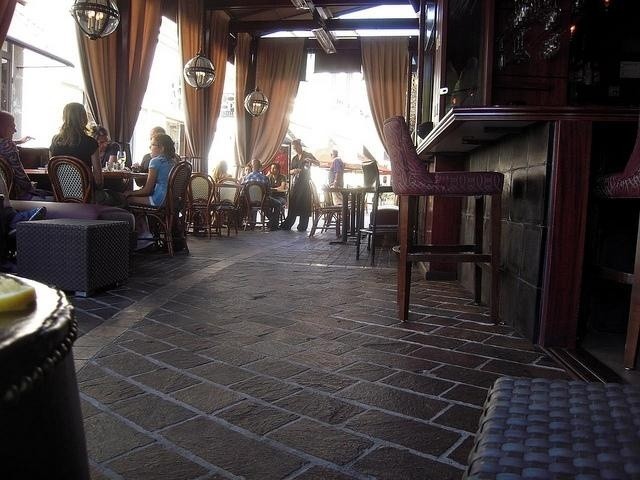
418 122 432 139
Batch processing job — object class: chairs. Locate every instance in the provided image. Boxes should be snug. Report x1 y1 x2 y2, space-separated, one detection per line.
47 155 94 205
0 156 15 200
589 126 639 372
129 161 399 266
382 115 505 324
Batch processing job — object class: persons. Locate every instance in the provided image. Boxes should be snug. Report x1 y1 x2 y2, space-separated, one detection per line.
242 159 269 232
212 161 233 183
48 102 128 210
0 111 55 202
279 139 320 232
265 163 286 231
86 125 120 170
121 133 180 251
327 149 344 219
140 126 166 171
244 164 253 176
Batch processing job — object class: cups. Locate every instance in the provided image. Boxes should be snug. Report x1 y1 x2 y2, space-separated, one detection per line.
106 150 127 173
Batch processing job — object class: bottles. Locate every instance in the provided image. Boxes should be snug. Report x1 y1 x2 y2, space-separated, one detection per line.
269 177 280 188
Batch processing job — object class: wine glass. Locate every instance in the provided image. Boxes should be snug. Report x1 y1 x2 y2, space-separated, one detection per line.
497 0 563 70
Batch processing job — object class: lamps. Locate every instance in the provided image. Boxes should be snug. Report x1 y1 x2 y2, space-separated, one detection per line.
183 50 215 91
70 0 121 40
291 0 337 54
243 85 269 117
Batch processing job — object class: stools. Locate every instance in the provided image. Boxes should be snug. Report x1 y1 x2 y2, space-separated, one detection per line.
461 375 639 479
0 274 90 480
14 218 131 298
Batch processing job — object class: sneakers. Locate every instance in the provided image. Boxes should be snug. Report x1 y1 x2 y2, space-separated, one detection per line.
134 238 155 251
8 207 47 237
